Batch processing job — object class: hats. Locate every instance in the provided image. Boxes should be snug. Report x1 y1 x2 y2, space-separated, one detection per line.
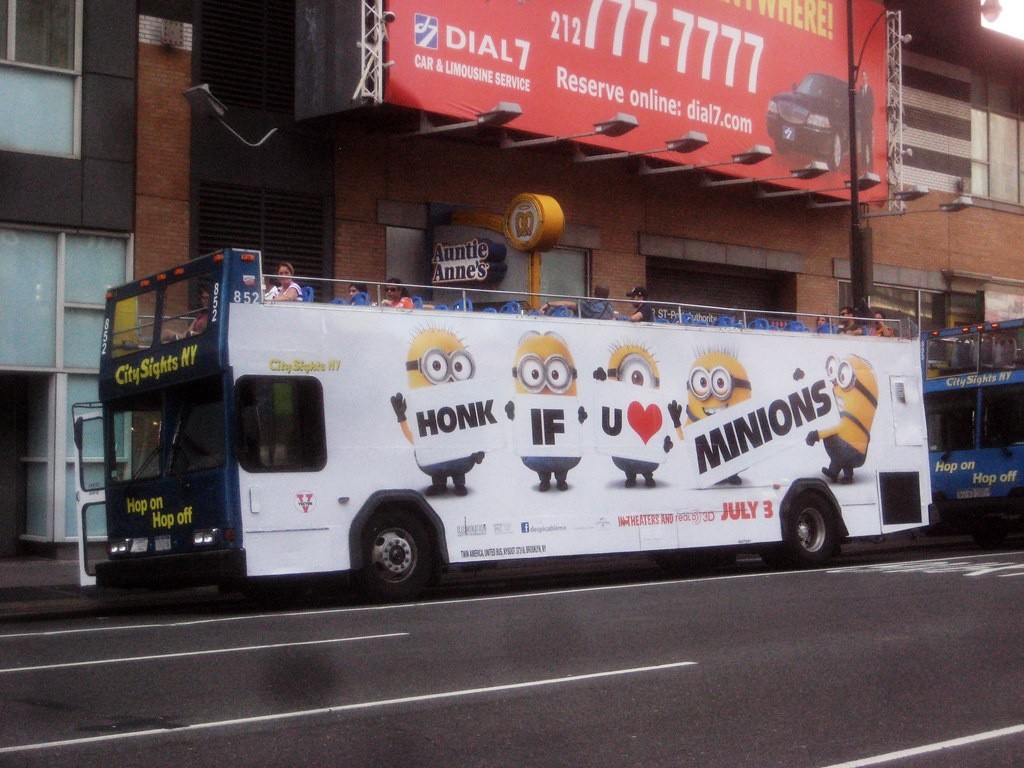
626 287 648 298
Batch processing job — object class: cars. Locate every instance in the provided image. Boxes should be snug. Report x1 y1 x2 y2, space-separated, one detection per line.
766 71 874 173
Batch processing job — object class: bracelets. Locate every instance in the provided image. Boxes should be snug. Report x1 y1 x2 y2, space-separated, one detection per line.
546 302 550 307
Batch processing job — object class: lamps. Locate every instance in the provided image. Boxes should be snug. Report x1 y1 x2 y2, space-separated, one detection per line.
430 102 522 135
900 34 912 44
557 112 638 140
380 11 394 23
887 11 895 21
694 145 772 168
629 130 708 155
900 148 913 157
807 172 880 192
905 196 973 214
181 84 277 147
754 162 828 182
867 185 928 202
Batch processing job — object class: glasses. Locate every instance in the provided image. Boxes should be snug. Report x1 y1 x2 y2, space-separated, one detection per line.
386 287 396 292
839 313 848 316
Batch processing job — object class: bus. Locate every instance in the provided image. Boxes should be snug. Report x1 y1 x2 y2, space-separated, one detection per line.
71 245 941 604
920 317 1024 549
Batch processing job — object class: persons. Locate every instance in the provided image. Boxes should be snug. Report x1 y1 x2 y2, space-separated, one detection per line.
163 288 210 341
874 312 895 337
540 283 614 321
345 276 369 296
625 286 654 322
383 278 414 310
859 70 875 173
816 313 830 326
837 306 863 335
264 261 303 301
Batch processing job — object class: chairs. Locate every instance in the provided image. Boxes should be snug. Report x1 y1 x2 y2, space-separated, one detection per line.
992 336 1018 370
947 336 973 372
978 335 994 370
291 288 867 336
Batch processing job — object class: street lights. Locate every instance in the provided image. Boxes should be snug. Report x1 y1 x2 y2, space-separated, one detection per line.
849 0 1002 319
